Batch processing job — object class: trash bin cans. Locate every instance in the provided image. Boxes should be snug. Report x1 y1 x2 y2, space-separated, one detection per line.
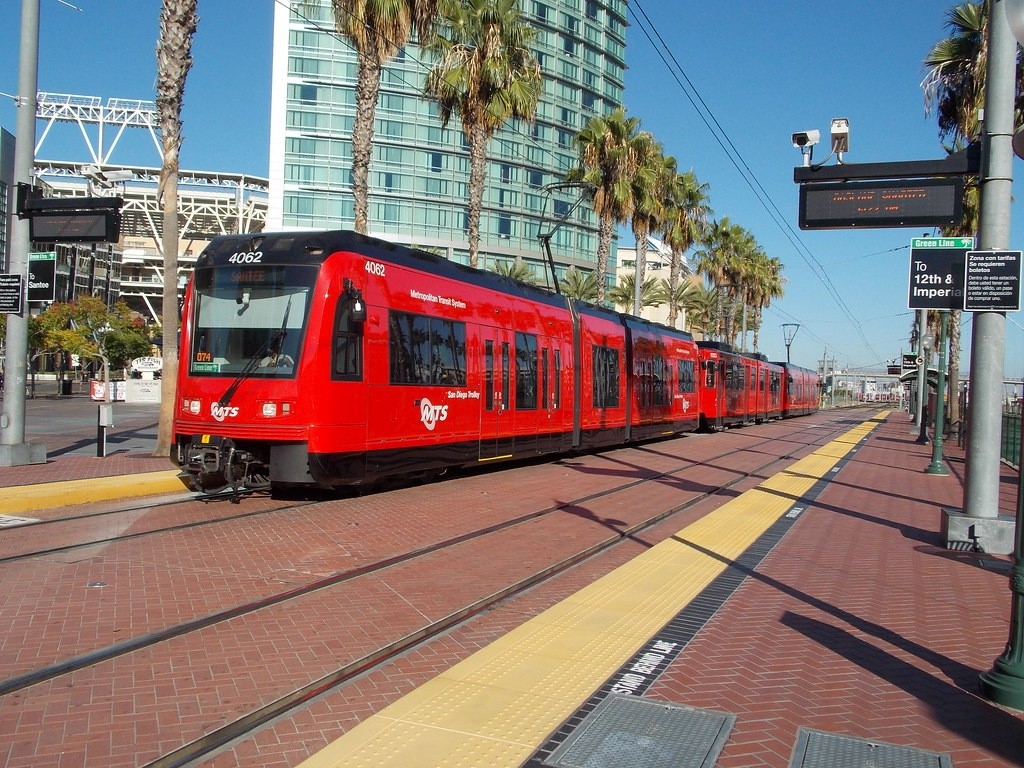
62 379 73 395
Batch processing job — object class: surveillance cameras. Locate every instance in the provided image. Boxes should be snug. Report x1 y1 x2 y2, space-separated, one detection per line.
830 118 850 152
791 130 820 147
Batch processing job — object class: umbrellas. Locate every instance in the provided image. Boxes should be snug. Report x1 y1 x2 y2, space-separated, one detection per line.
149 331 204 347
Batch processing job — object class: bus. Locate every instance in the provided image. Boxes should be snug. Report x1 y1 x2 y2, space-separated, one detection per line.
695 323 786 433
161 228 707 506
769 322 820 419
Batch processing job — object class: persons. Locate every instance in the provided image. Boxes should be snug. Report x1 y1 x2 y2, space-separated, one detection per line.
259 343 294 368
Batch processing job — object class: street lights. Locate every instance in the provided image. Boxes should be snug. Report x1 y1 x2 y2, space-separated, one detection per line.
914 327 935 443
